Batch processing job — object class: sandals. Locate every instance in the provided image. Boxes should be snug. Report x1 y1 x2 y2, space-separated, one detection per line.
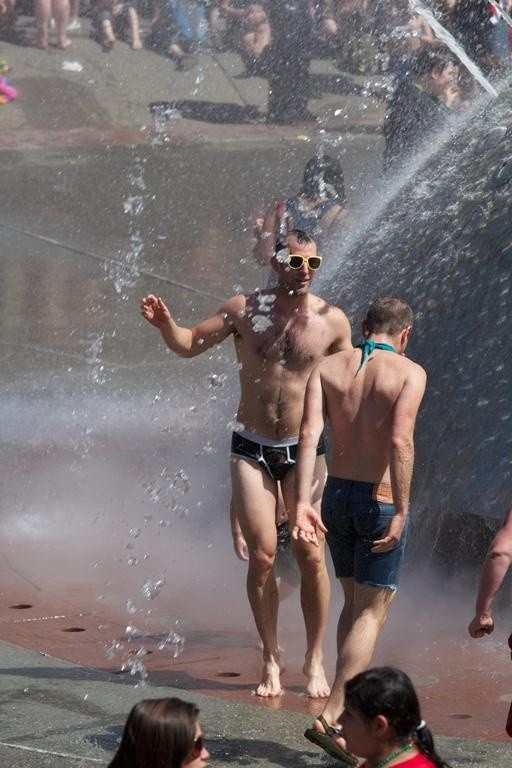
305 716 359 765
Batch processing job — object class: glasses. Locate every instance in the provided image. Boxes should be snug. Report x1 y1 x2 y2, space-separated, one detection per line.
287 255 323 271
192 736 207 757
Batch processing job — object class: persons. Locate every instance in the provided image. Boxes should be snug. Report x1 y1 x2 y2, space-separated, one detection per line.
106 697 212 768
287 297 427 767
251 156 355 291
138 227 356 700
336 665 449 767
230 476 300 653
2 1 512 174
467 507 512 738
